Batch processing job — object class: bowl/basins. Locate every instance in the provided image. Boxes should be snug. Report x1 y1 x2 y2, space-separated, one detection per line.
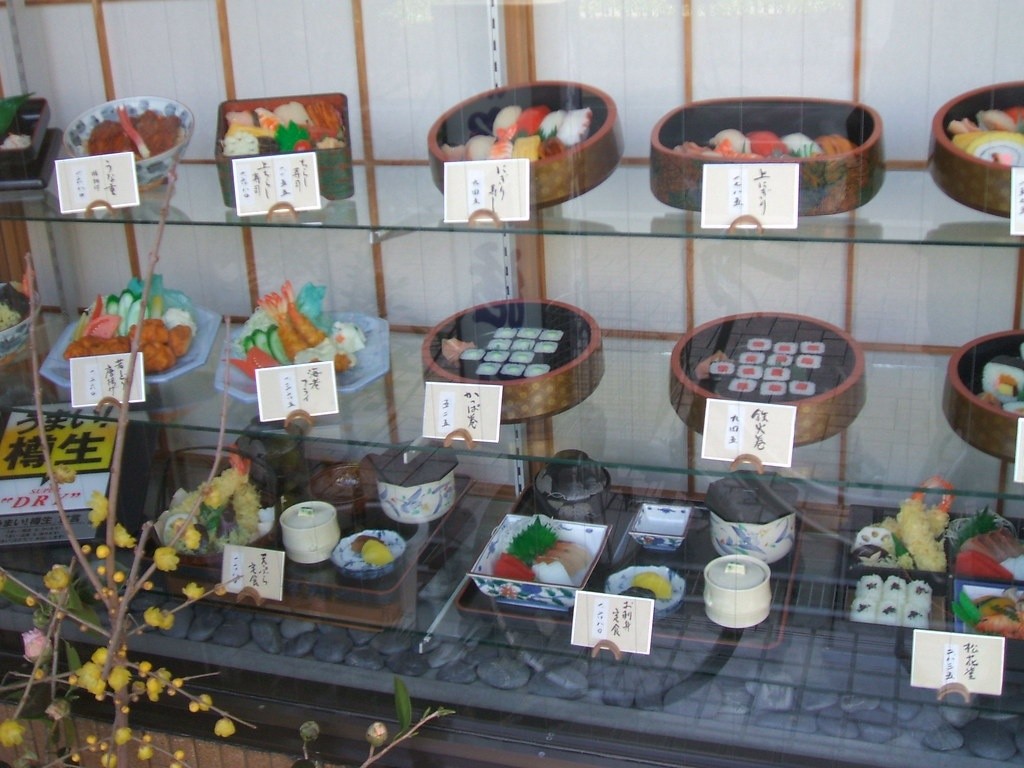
533 449 610 522
465 513 614 611
0 283 41 356
215 93 356 207
308 466 375 512
630 502 692 551
707 512 796 566
650 97 885 217
428 81 625 211
943 329 1024 463
422 297 605 425
930 81 1024 217
63 97 194 190
670 312 866 449
237 417 306 488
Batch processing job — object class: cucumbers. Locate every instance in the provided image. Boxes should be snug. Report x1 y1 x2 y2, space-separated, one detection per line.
106 289 149 335
244 325 292 365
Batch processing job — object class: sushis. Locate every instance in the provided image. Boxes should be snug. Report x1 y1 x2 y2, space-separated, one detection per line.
534 541 595 581
946 106 1024 168
977 341 1024 417
972 594 1024 638
457 327 566 379
618 572 672 604
708 127 858 159
439 103 593 162
850 574 933 628
351 534 393 565
706 337 827 402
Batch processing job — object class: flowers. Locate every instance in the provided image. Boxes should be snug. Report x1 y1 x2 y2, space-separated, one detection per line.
0 119 458 766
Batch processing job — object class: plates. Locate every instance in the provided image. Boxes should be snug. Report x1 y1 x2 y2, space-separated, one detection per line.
215 311 390 402
604 565 686 618
332 530 406 580
39 304 221 389
954 579 1024 633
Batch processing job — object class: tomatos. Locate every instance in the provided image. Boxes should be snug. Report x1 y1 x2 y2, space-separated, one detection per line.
83 315 121 338
247 346 282 373
295 139 312 151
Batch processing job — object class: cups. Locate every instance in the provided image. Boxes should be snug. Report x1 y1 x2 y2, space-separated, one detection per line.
703 555 771 628
279 502 340 565
376 442 457 524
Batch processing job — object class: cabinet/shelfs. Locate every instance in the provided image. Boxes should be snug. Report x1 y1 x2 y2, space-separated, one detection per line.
0 1 1024 768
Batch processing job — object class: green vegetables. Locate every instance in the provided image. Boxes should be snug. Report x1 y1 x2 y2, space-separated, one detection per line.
274 121 309 153
507 516 558 565
953 505 999 550
